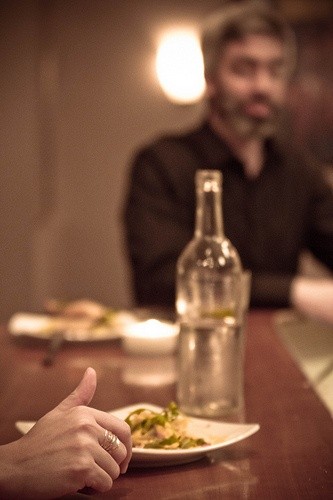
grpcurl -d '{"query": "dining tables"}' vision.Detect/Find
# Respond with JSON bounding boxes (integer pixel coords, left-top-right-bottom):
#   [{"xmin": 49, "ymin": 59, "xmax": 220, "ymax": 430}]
[{"xmin": 0, "ymin": 305, "xmax": 333, "ymax": 500}]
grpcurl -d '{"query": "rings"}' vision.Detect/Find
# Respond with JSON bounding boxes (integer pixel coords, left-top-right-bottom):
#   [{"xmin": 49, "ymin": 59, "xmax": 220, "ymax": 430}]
[{"xmin": 100, "ymin": 430, "xmax": 120, "ymax": 453}]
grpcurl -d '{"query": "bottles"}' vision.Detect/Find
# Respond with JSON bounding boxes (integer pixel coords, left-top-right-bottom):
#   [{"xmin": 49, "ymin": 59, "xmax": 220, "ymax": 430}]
[{"xmin": 175, "ymin": 169, "xmax": 244, "ymax": 427}]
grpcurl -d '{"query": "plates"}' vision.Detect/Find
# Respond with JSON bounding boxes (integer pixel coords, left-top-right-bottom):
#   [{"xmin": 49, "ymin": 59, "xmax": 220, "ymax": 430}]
[
  {"xmin": 8, "ymin": 313, "xmax": 120, "ymax": 343},
  {"xmin": 14, "ymin": 402, "xmax": 260, "ymax": 469}
]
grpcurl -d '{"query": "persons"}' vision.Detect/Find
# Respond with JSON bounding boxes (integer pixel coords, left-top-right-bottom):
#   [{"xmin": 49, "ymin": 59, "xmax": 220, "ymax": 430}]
[
  {"xmin": 0, "ymin": 366, "xmax": 132, "ymax": 500},
  {"xmin": 121, "ymin": 0, "xmax": 333, "ymax": 323}
]
